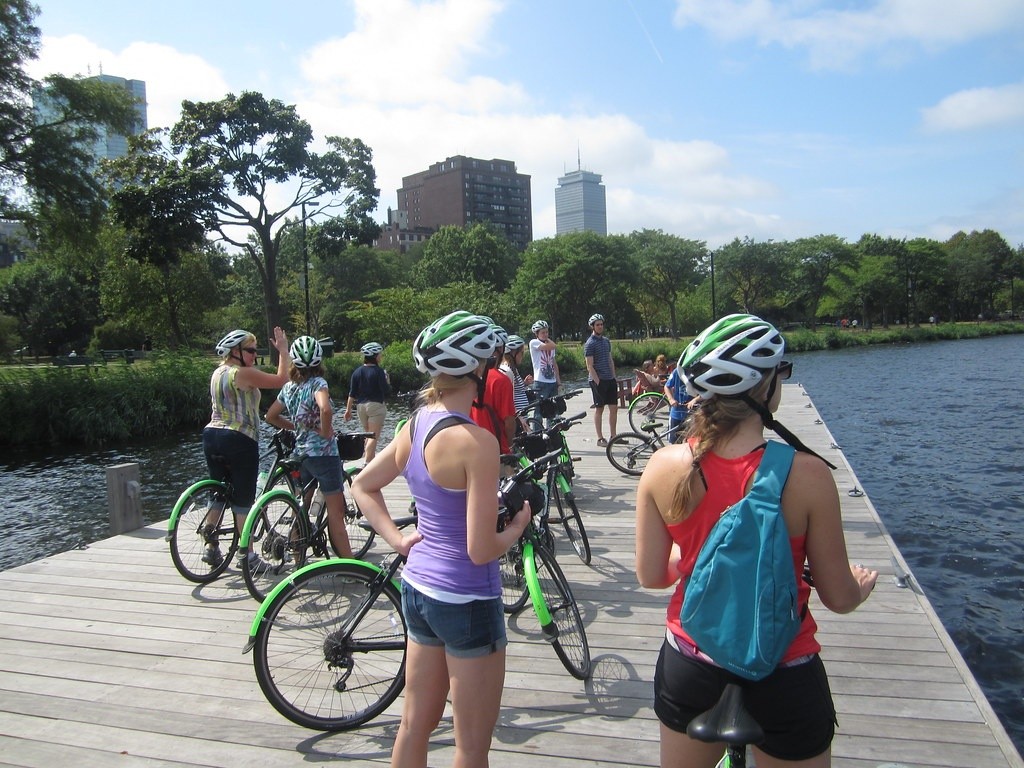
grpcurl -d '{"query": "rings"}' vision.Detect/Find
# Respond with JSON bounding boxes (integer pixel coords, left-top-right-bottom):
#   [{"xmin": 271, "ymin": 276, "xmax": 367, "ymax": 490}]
[{"xmin": 855, "ymin": 563, "xmax": 863, "ymax": 568}]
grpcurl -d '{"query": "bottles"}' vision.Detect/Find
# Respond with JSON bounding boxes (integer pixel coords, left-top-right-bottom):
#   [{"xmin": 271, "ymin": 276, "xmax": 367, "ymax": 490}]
[
  {"xmin": 537, "ymin": 482, "xmax": 548, "ymax": 517},
  {"xmin": 254, "ymin": 472, "xmax": 267, "ymax": 502},
  {"xmin": 310, "ymin": 485, "xmax": 325, "ymax": 516}
]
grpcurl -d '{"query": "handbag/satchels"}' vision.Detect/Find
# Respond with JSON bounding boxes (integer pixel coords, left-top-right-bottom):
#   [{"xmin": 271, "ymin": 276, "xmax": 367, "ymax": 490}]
[{"xmin": 679, "ymin": 439, "xmax": 807, "ymax": 681}]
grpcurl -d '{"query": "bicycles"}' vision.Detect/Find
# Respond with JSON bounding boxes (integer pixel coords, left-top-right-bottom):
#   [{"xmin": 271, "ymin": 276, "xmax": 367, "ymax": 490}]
[
  {"xmin": 686, "ymin": 561, "xmax": 876, "ymax": 768},
  {"xmin": 165, "ymin": 416, "xmax": 303, "ymax": 585},
  {"xmin": 627, "ymin": 373, "xmax": 673, "ymax": 439},
  {"xmin": 606, "ymin": 402, "xmax": 702, "ymax": 476},
  {"xmin": 236, "ymin": 430, "xmax": 377, "ymax": 605},
  {"xmin": 496, "ymin": 390, "xmax": 592, "ymax": 613},
  {"xmin": 241, "ymin": 446, "xmax": 592, "ymax": 733}
]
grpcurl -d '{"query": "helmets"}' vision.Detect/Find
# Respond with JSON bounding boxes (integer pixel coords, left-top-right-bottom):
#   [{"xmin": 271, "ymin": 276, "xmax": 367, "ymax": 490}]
[
  {"xmin": 532, "ymin": 320, "xmax": 548, "ymax": 333},
  {"xmin": 215, "ymin": 330, "xmax": 248, "ymax": 356},
  {"xmin": 505, "ymin": 334, "xmax": 525, "ymax": 349},
  {"xmin": 491, "ymin": 326, "xmax": 507, "ymax": 347},
  {"xmin": 588, "ymin": 314, "xmax": 604, "ymax": 327},
  {"xmin": 289, "ymin": 335, "xmax": 323, "ymax": 368},
  {"xmin": 412, "ymin": 310, "xmax": 495, "ymax": 376},
  {"xmin": 677, "ymin": 313, "xmax": 784, "ymax": 398},
  {"xmin": 360, "ymin": 342, "xmax": 383, "ymax": 356}
]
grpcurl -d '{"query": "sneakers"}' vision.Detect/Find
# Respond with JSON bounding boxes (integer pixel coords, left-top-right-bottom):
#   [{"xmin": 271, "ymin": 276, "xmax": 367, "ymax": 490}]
[
  {"xmin": 236, "ymin": 552, "xmax": 272, "ymax": 572},
  {"xmin": 597, "ymin": 439, "xmax": 609, "ymax": 447},
  {"xmin": 609, "ymin": 437, "xmax": 628, "ymax": 444},
  {"xmin": 201, "ymin": 548, "xmax": 223, "ymax": 565}
]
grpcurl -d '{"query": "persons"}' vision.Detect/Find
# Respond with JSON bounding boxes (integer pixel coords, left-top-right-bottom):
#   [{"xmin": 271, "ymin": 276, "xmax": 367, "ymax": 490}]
[
  {"xmin": 634, "ymin": 312, "xmax": 878, "ymax": 768},
  {"xmin": 200, "ymin": 327, "xmax": 291, "ymax": 573},
  {"xmin": 928, "ymin": 315, "xmax": 934, "ymax": 325},
  {"xmin": 350, "ymin": 309, "xmax": 531, "ymax": 768},
  {"xmin": 528, "ymin": 320, "xmax": 563, "ymax": 434},
  {"xmin": 852, "ymin": 318, "xmax": 858, "ymax": 328},
  {"xmin": 583, "ymin": 314, "xmax": 629, "ymax": 446},
  {"xmin": 836, "ymin": 318, "xmax": 850, "ymax": 329},
  {"xmin": 343, "ymin": 341, "xmax": 391, "ymax": 469},
  {"xmin": 263, "ymin": 336, "xmax": 372, "ymax": 584},
  {"xmin": 643, "ymin": 354, "xmax": 675, "ymax": 393},
  {"xmin": 663, "ymin": 368, "xmax": 704, "ymax": 446},
  {"xmin": 469, "ymin": 326, "xmax": 552, "ymax": 560}
]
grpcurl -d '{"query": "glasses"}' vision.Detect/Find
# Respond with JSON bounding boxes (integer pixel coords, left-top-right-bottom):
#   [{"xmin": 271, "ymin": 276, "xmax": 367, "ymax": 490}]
[
  {"xmin": 776, "ymin": 361, "xmax": 793, "ymax": 379},
  {"xmin": 238, "ymin": 348, "xmax": 256, "ymax": 354}
]
